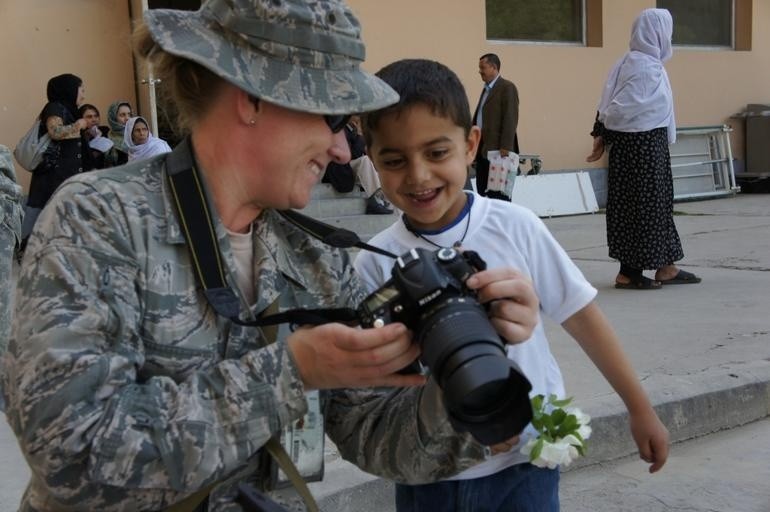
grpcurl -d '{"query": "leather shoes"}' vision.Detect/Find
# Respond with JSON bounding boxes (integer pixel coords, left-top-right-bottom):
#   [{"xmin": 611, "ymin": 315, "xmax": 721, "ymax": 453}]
[{"xmin": 366, "ymin": 204, "xmax": 393, "ymax": 214}]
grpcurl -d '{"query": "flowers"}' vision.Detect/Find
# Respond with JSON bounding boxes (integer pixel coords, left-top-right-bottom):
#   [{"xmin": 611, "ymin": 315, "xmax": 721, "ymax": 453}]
[{"xmin": 512, "ymin": 390, "xmax": 594, "ymax": 472}]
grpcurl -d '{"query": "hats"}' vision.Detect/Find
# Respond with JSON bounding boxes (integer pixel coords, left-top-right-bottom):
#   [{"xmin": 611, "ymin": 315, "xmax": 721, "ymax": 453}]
[{"xmin": 143, "ymin": 1, "xmax": 400, "ymax": 116}]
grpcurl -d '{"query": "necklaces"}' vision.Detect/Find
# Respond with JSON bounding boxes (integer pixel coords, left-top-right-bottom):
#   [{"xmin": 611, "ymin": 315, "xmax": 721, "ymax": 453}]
[{"xmin": 404, "ymin": 192, "xmax": 472, "ymax": 249}]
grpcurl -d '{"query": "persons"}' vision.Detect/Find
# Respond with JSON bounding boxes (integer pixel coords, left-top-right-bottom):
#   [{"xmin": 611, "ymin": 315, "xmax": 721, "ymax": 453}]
[
  {"xmin": 79, "ymin": 101, "xmax": 171, "ymax": 173},
  {"xmin": 28, "ymin": 73, "xmax": 91, "ymax": 217},
  {"xmin": 350, "ymin": 55, "xmax": 670, "ymax": 512},
  {"xmin": 322, "ymin": 115, "xmax": 394, "ymax": 216},
  {"xmin": 586, "ymin": 7, "xmax": 702, "ymax": 289},
  {"xmin": 3, "ymin": 1, "xmax": 540, "ymax": 512},
  {"xmin": 472, "ymin": 53, "xmax": 521, "ymax": 203}
]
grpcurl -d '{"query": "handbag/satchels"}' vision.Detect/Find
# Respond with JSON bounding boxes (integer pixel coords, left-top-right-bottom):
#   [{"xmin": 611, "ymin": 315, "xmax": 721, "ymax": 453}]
[
  {"xmin": 322, "ymin": 161, "xmax": 354, "ymax": 193},
  {"xmin": 13, "ymin": 117, "xmax": 51, "ymax": 171},
  {"xmin": 487, "ymin": 149, "xmax": 520, "ymax": 197}
]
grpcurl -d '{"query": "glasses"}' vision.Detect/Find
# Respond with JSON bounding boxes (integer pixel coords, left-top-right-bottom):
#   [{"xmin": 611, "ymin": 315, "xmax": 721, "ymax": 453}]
[{"xmin": 324, "ymin": 113, "xmax": 350, "ymax": 134}]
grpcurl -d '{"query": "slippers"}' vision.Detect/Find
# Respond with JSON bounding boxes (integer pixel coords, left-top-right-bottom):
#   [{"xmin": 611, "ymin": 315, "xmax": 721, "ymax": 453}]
[
  {"xmin": 614, "ymin": 277, "xmax": 662, "ymax": 289},
  {"xmin": 661, "ymin": 270, "xmax": 701, "ymax": 284}
]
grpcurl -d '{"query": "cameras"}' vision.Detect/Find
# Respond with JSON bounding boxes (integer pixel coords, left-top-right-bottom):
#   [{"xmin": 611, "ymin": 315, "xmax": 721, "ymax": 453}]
[{"xmin": 352, "ymin": 248, "xmax": 533, "ymax": 446}]
